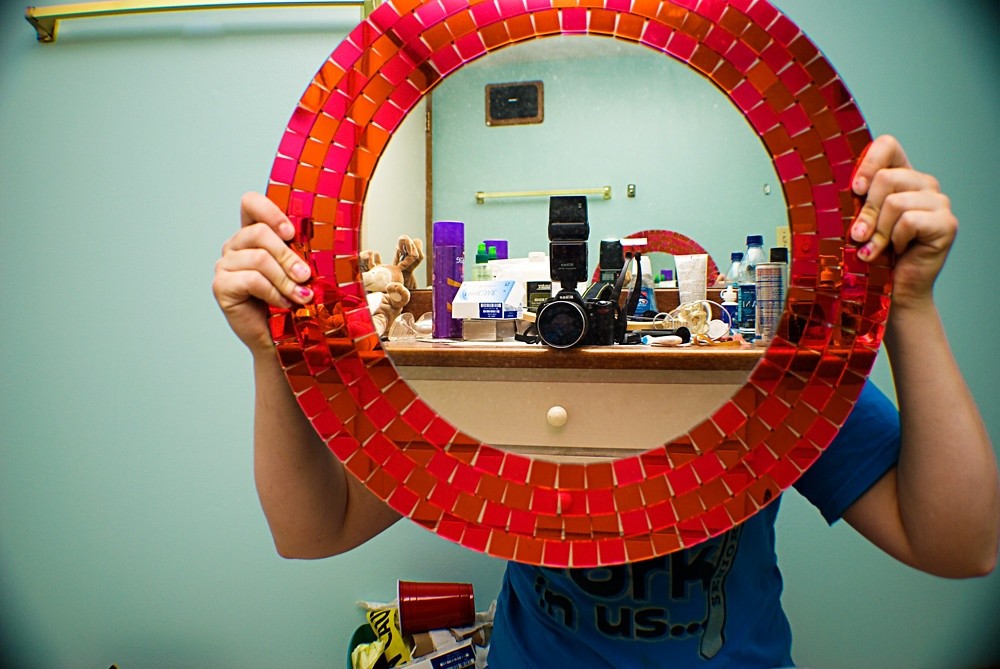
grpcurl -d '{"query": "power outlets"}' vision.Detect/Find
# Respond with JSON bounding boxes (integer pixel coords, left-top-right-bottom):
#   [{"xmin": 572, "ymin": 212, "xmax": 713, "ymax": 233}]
[{"xmin": 777, "ymin": 226, "xmax": 792, "ymax": 251}]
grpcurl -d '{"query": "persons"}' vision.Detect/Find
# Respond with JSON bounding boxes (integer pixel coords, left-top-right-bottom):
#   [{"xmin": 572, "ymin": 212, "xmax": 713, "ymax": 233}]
[{"xmin": 213, "ymin": 136, "xmax": 1000, "ymax": 669}]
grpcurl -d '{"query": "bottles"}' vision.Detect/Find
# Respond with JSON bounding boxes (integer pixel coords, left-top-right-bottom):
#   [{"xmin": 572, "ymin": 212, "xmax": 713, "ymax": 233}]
[
  {"xmin": 627, "ymin": 256, "xmax": 658, "ymax": 317},
  {"xmin": 654, "ymin": 269, "xmax": 673, "ymax": 288},
  {"xmin": 471, "ymin": 240, "xmax": 509, "ymax": 281},
  {"xmin": 432, "ymin": 222, "xmax": 465, "ymax": 339},
  {"xmin": 525, "ymin": 252, "xmax": 552, "ymax": 313},
  {"xmin": 721, "ymin": 251, "xmax": 744, "ymax": 336},
  {"xmin": 738, "ymin": 235, "xmax": 768, "ymax": 343}
]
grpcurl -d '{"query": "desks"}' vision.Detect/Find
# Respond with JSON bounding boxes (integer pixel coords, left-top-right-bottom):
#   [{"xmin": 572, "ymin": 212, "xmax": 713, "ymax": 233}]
[{"xmin": 380, "ymin": 291, "xmax": 769, "ymax": 462}]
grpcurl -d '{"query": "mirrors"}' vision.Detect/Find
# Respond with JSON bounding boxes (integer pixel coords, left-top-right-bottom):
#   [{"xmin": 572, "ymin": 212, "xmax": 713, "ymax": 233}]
[
  {"xmin": 355, "ymin": 32, "xmax": 789, "ymax": 289},
  {"xmin": 263, "ymin": 0, "xmax": 896, "ymax": 569},
  {"xmin": 592, "ymin": 230, "xmax": 720, "ymax": 288}
]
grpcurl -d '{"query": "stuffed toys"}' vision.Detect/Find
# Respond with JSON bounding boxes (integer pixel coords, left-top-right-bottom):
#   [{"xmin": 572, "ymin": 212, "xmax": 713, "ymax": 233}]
[{"xmin": 358, "ymin": 236, "xmax": 420, "ymax": 340}]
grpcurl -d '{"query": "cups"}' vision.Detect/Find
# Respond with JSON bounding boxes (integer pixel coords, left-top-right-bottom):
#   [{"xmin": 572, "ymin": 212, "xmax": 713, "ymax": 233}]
[{"xmin": 396, "ymin": 579, "xmax": 475, "ymax": 638}]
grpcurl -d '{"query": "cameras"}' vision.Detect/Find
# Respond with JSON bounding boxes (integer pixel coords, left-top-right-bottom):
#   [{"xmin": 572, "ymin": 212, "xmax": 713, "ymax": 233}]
[{"xmin": 535, "ymin": 196, "xmax": 623, "ymax": 349}]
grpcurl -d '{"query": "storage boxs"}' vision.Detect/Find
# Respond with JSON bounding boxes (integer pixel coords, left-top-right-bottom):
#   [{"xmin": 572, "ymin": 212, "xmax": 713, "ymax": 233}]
[
  {"xmin": 452, "ymin": 280, "xmax": 519, "ymax": 320},
  {"xmin": 389, "ymin": 637, "xmax": 477, "ymax": 669}
]
